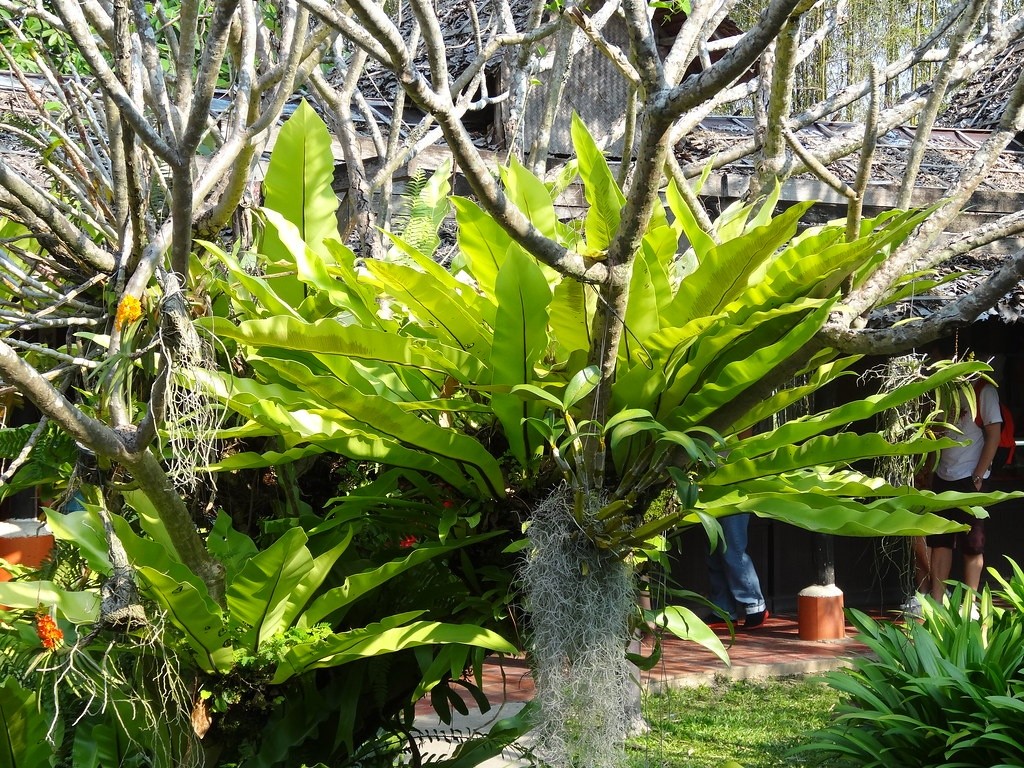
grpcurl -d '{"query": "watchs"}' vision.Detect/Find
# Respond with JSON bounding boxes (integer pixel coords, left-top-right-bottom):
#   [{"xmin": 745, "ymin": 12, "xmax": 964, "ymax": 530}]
[{"xmin": 972, "ymin": 474, "xmax": 982, "ymax": 483}]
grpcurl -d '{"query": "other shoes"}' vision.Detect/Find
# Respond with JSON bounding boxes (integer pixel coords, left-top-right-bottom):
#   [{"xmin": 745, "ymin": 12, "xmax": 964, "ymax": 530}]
[
  {"xmin": 899, "ymin": 594, "xmax": 926, "ymax": 609},
  {"xmin": 702, "ymin": 613, "xmax": 738, "ymax": 628},
  {"xmin": 743, "ymin": 609, "xmax": 769, "ymax": 630},
  {"xmin": 909, "ymin": 589, "xmax": 952, "ymax": 615}
]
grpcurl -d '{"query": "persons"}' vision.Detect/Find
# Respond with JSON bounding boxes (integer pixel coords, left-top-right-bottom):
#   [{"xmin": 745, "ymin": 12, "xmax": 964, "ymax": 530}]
[
  {"xmin": 930, "ymin": 337, "xmax": 1003, "ymax": 622},
  {"xmin": 701, "ymin": 425, "xmax": 769, "ymax": 630},
  {"xmin": 900, "ymin": 536, "xmax": 931, "ymax": 617}
]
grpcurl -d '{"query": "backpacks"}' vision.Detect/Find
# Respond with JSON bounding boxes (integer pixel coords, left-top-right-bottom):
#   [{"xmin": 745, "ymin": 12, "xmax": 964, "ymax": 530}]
[{"xmin": 974, "ymin": 379, "xmax": 1015, "ymax": 472}]
